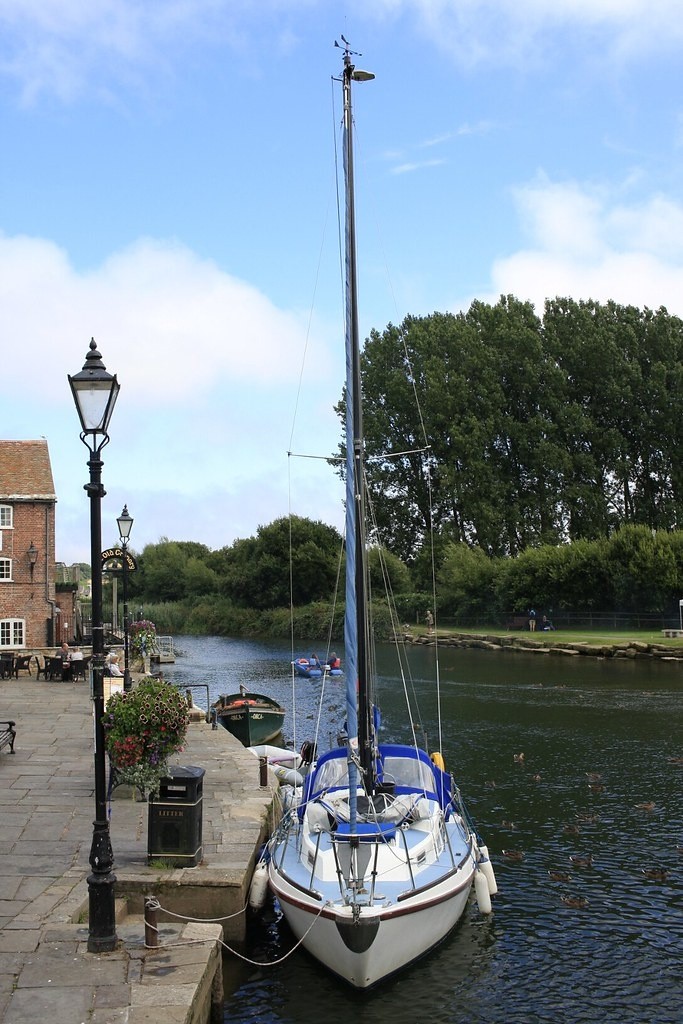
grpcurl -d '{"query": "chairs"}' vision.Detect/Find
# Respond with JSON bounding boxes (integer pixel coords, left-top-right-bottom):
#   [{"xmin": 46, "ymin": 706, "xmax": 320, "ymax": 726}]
[
  {"xmin": 35, "ymin": 655, "xmax": 93, "ymax": 683},
  {"xmin": 0, "ymin": 653, "xmax": 33, "ymax": 679}
]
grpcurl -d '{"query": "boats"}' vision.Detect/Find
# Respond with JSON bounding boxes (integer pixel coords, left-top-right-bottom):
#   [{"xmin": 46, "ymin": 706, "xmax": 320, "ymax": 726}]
[
  {"xmin": 291, "ymin": 657, "xmax": 323, "ymax": 678},
  {"xmin": 211, "ymin": 685, "xmax": 287, "ymax": 746},
  {"xmin": 319, "ymin": 660, "xmax": 343, "ymax": 675}
]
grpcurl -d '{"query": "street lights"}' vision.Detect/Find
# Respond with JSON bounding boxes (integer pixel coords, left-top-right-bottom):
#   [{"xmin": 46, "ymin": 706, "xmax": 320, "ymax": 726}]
[
  {"xmin": 114, "ymin": 504, "xmax": 133, "ymax": 692},
  {"xmin": 66, "ymin": 335, "xmax": 122, "ymax": 953}
]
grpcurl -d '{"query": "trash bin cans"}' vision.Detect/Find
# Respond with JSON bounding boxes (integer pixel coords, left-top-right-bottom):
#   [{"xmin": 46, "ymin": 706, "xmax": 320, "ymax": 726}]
[{"xmin": 146, "ymin": 765, "xmax": 206, "ymax": 868}]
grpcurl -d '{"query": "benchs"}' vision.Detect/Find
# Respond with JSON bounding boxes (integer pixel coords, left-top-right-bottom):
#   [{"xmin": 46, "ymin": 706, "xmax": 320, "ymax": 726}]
[{"xmin": 0, "ymin": 721, "xmax": 16, "ymax": 754}]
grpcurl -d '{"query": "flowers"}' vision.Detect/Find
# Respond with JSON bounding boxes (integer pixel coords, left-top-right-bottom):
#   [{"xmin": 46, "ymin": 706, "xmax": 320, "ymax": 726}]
[
  {"xmin": 99, "ymin": 676, "xmax": 191, "ymax": 802},
  {"xmin": 129, "ymin": 619, "xmax": 156, "ymax": 647}
]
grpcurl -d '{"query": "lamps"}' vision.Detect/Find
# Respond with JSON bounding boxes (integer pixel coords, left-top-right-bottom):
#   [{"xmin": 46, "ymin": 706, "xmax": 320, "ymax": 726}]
[{"xmin": 27, "ymin": 541, "xmax": 39, "ymax": 568}]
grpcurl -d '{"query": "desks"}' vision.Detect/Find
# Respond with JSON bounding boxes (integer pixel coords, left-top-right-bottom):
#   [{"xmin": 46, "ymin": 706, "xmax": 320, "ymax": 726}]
[
  {"xmin": 0, "ymin": 658, "xmax": 12, "ymax": 678},
  {"xmin": 61, "ymin": 660, "xmax": 74, "ymax": 683}
]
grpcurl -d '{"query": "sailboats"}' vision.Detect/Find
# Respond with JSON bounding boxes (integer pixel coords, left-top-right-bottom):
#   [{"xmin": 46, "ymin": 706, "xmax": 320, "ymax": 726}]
[{"xmin": 261, "ymin": 33, "xmax": 497, "ymax": 989}]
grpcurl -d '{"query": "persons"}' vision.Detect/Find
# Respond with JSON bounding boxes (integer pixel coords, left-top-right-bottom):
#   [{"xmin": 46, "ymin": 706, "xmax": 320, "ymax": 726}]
[
  {"xmin": 425, "ymin": 611, "xmax": 434, "ymax": 635},
  {"xmin": 324, "ymin": 652, "xmax": 340, "ymax": 670},
  {"xmin": 527, "ymin": 607, "xmax": 536, "ymax": 631},
  {"xmin": 56, "ymin": 643, "xmax": 83, "ymax": 661},
  {"xmin": 105, "ymin": 653, "xmax": 135, "ymax": 682},
  {"xmin": 309, "ymin": 654, "xmax": 322, "ymax": 670}
]
